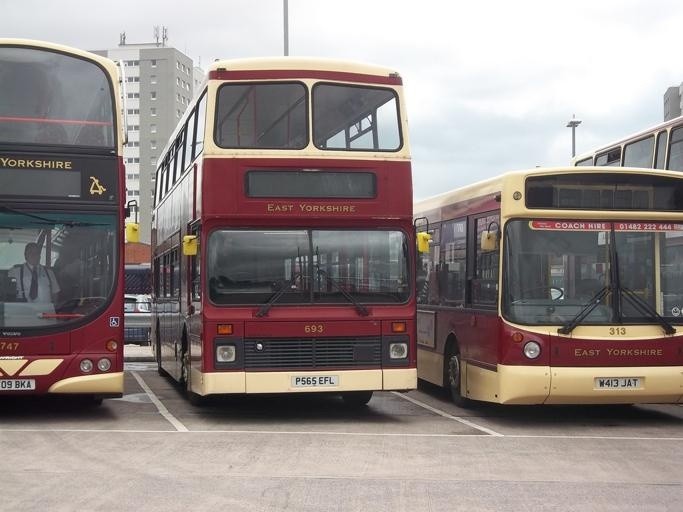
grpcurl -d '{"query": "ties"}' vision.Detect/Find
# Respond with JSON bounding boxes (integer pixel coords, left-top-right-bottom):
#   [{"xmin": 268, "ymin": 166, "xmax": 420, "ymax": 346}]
[{"xmin": 29, "ymin": 266, "xmax": 38, "ymax": 299}]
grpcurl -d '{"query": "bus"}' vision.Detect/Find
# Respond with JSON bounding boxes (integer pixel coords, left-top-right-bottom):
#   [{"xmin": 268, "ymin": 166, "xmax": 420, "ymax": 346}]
[
  {"xmin": 1, "ymin": 37, "xmax": 140, "ymax": 406},
  {"xmin": 563, "ymin": 116, "xmax": 683, "ymax": 310},
  {"xmin": 149, "ymin": 56, "xmax": 432, "ymax": 406},
  {"xmin": 392, "ymin": 165, "xmax": 682, "ymax": 409}
]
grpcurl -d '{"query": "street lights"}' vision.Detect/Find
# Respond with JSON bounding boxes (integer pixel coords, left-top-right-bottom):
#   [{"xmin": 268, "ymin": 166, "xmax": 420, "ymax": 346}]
[{"xmin": 565, "ymin": 114, "xmax": 582, "ymax": 158}]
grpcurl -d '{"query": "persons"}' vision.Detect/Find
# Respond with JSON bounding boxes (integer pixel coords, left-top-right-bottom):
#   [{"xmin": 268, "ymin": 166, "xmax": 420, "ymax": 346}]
[{"xmin": 4, "ymin": 242, "xmax": 64, "ymax": 310}]
